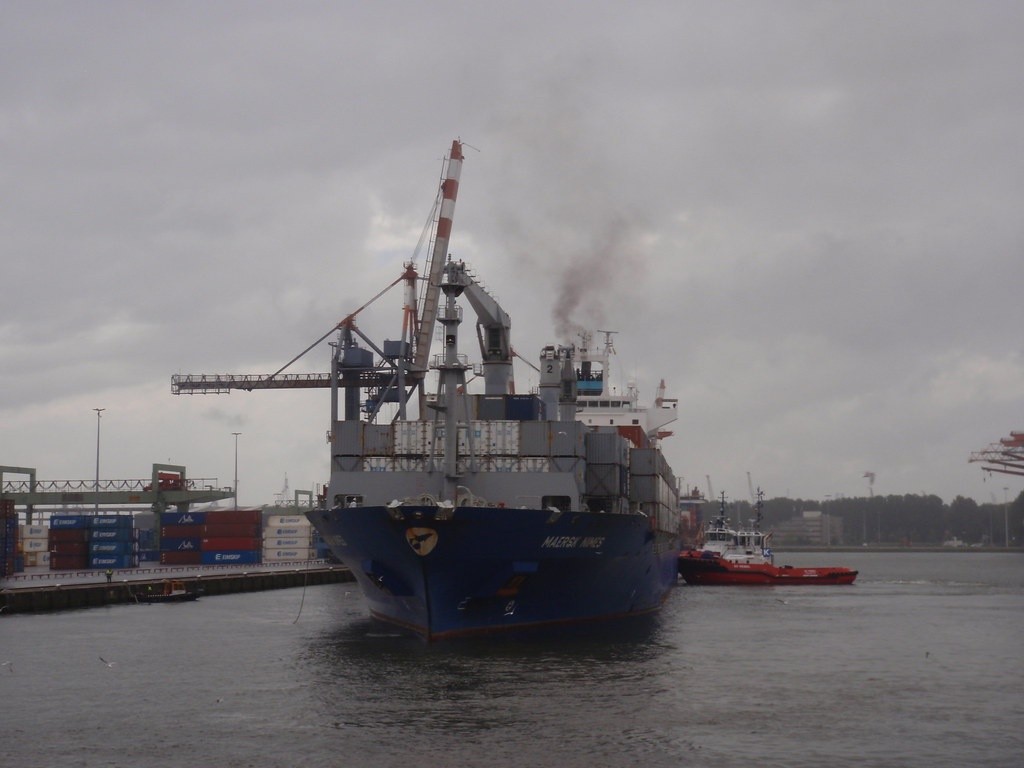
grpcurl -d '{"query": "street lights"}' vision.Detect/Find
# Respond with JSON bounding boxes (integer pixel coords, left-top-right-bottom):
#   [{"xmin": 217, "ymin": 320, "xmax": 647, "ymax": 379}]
[
  {"xmin": 232, "ymin": 431, "xmax": 242, "ymax": 511},
  {"xmin": 824, "ymin": 494, "xmax": 832, "ymax": 546},
  {"xmin": 93, "ymin": 407, "xmax": 107, "ymax": 516},
  {"xmin": 1003, "ymin": 487, "xmax": 1010, "ymax": 551}
]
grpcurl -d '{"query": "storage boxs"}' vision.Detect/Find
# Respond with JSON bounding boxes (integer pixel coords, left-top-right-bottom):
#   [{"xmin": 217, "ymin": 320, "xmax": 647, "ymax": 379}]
[
  {"xmin": 0, "ymin": 498, "xmax": 329, "ymax": 575},
  {"xmin": 331, "ymin": 395, "xmax": 680, "ymax": 533}
]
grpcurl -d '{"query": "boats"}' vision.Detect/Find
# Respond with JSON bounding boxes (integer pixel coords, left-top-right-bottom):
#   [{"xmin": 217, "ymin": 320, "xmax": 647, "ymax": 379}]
[
  {"xmin": 699, "ymin": 489, "xmax": 738, "ymax": 559},
  {"xmin": 144, "ymin": 582, "xmax": 200, "ymax": 604},
  {"xmin": 301, "ymin": 260, "xmax": 690, "ymax": 644},
  {"xmin": 721, "ymin": 485, "xmax": 775, "ymax": 567},
  {"xmin": 677, "ymin": 548, "xmax": 860, "ymax": 586}
]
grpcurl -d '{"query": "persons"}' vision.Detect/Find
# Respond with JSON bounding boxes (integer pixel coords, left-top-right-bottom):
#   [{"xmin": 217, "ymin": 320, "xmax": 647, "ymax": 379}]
[
  {"xmin": 146, "ymin": 585, "xmax": 152, "ymax": 594},
  {"xmin": 105, "ymin": 568, "xmax": 113, "ymax": 583},
  {"xmin": 349, "ymin": 498, "xmax": 356, "ymax": 507}
]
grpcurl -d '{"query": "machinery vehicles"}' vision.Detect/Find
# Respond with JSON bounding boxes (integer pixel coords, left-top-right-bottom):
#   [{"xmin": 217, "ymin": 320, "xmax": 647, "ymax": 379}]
[{"xmin": 171, "ymin": 139, "xmax": 548, "ymax": 421}]
